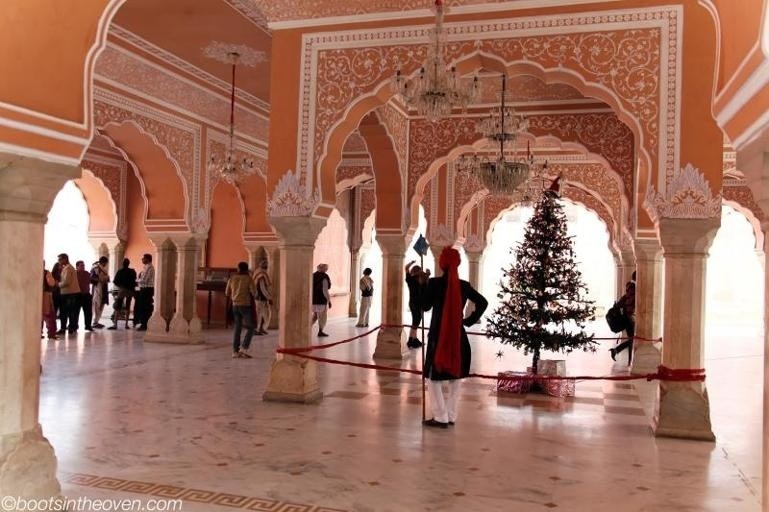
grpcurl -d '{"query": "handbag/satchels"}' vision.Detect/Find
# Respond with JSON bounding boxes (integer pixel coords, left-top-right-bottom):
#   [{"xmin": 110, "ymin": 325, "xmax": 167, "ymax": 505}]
[
  {"xmin": 113, "ymin": 298, "xmax": 122, "ymax": 310},
  {"xmin": 90, "ymin": 272, "xmax": 99, "ymax": 284}
]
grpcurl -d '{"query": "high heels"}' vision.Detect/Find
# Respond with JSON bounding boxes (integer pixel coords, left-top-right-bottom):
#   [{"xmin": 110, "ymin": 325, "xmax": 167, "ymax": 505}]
[{"xmin": 608, "ymin": 347, "xmax": 616, "ymax": 362}]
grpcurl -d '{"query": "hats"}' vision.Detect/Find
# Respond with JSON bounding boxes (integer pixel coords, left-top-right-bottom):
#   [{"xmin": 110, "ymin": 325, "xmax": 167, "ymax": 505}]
[
  {"xmin": 259, "ymin": 260, "xmax": 268, "ymax": 267},
  {"xmin": 317, "ymin": 264, "xmax": 328, "ymax": 272}
]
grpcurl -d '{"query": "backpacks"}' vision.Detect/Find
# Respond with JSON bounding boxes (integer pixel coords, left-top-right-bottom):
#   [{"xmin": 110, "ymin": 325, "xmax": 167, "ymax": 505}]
[{"xmin": 606, "ymin": 295, "xmax": 628, "ymax": 333}]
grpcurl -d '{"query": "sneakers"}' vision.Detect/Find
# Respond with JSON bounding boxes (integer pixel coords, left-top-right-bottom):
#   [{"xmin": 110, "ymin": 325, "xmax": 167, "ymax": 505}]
[
  {"xmin": 41, "ymin": 322, "xmax": 149, "ymax": 339},
  {"xmin": 254, "ymin": 329, "xmax": 268, "ymax": 335},
  {"xmin": 239, "ymin": 347, "xmax": 253, "ymax": 358},
  {"xmin": 231, "ymin": 351, "xmax": 241, "ymax": 358}
]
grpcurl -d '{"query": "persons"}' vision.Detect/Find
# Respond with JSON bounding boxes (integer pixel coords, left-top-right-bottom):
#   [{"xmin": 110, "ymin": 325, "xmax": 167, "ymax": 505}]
[
  {"xmin": 416, "ymin": 245, "xmax": 488, "ymax": 431},
  {"xmin": 606, "ymin": 270, "xmax": 636, "ymax": 367},
  {"xmin": 57, "ymin": 253, "xmax": 82, "ymax": 334},
  {"xmin": 224, "ymin": 260, "xmax": 258, "ymax": 360},
  {"xmin": 133, "ymin": 252, "xmax": 156, "ymax": 328},
  {"xmin": 41, "ymin": 259, "xmax": 60, "ymax": 339},
  {"xmin": 311, "ymin": 262, "xmax": 333, "ymax": 338},
  {"xmin": 107, "ymin": 257, "xmax": 137, "ymax": 331},
  {"xmin": 252, "ymin": 258, "xmax": 275, "ymax": 336},
  {"xmin": 404, "ymin": 258, "xmax": 431, "ymax": 349},
  {"xmin": 74, "ymin": 260, "xmax": 96, "ymax": 331},
  {"xmin": 51, "ymin": 263, "xmax": 62, "ymax": 315},
  {"xmin": 88, "ymin": 256, "xmax": 112, "ymax": 329},
  {"xmin": 355, "ymin": 267, "xmax": 374, "ymax": 329}
]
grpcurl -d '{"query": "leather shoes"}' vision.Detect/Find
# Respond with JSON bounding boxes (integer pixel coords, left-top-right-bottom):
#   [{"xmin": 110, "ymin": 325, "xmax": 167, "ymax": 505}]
[
  {"xmin": 424, "ymin": 418, "xmax": 448, "ymax": 428},
  {"xmin": 318, "ymin": 332, "xmax": 328, "ymax": 336},
  {"xmin": 407, "ymin": 336, "xmax": 425, "ymax": 348}
]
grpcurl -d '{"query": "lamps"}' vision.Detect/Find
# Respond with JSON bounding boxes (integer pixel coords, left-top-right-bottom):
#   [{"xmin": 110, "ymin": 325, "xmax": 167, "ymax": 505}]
[
  {"xmin": 208, "ymin": 50, "xmax": 257, "ymax": 184},
  {"xmin": 391, "ymin": 0, "xmax": 483, "ymax": 127},
  {"xmin": 456, "ymin": 65, "xmax": 548, "ymax": 197}
]
